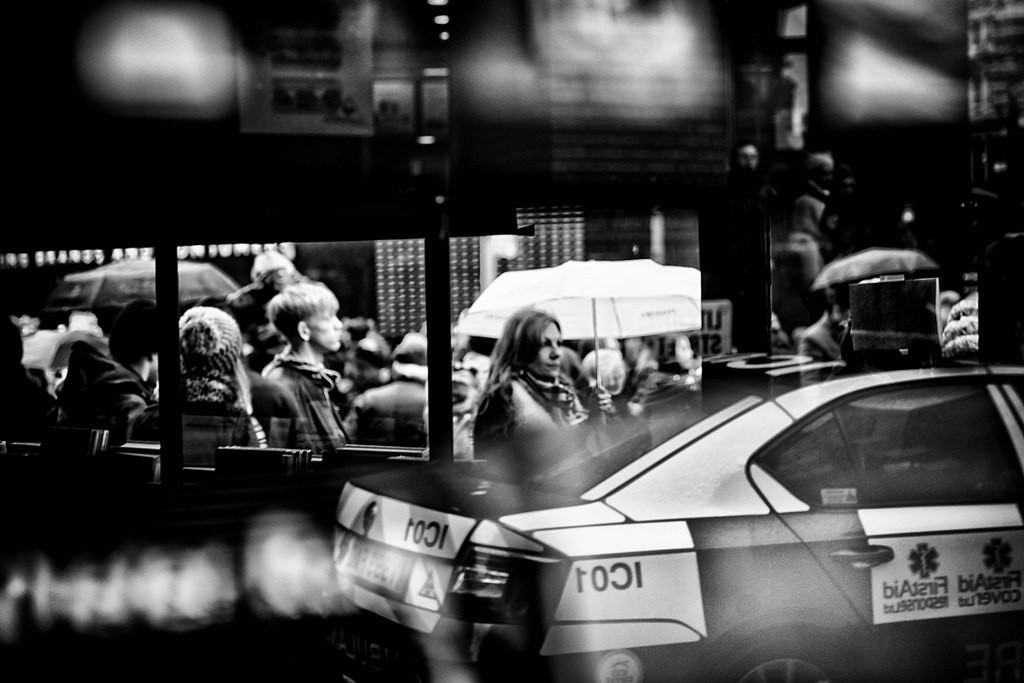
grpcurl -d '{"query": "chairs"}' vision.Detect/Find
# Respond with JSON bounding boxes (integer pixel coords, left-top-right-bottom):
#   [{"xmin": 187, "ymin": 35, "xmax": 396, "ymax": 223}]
[{"xmin": 886, "ymin": 407, "xmax": 979, "ymax": 502}]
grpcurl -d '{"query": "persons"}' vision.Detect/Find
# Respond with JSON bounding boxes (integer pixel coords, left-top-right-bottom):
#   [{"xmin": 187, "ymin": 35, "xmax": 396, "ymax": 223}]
[
  {"xmin": 1, "ymin": 250, "xmax": 705, "ymax": 470},
  {"xmin": 743, "ymin": 147, "xmax": 979, "ymax": 391}
]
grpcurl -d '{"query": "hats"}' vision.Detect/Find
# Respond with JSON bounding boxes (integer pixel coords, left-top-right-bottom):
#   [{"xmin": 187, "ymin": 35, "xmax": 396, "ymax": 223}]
[
  {"xmin": 179, "ymin": 305, "xmax": 241, "ymax": 372},
  {"xmin": 252, "ymin": 251, "xmax": 293, "ymax": 281}
]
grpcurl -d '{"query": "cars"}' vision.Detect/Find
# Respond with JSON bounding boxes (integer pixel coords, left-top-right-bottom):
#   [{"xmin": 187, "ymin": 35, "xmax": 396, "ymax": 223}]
[{"xmin": 330, "ymin": 361, "xmax": 1023, "ymax": 683}]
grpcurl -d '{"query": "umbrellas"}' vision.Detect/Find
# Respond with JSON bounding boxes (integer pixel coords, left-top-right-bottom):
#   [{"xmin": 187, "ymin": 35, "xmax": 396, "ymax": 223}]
[
  {"xmin": 45, "ymin": 261, "xmax": 241, "ymax": 312},
  {"xmin": 459, "ymin": 258, "xmax": 700, "ymax": 389},
  {"xmin": 810, "ymin": 247, "xmax": 937, "ymax": 291}
]
